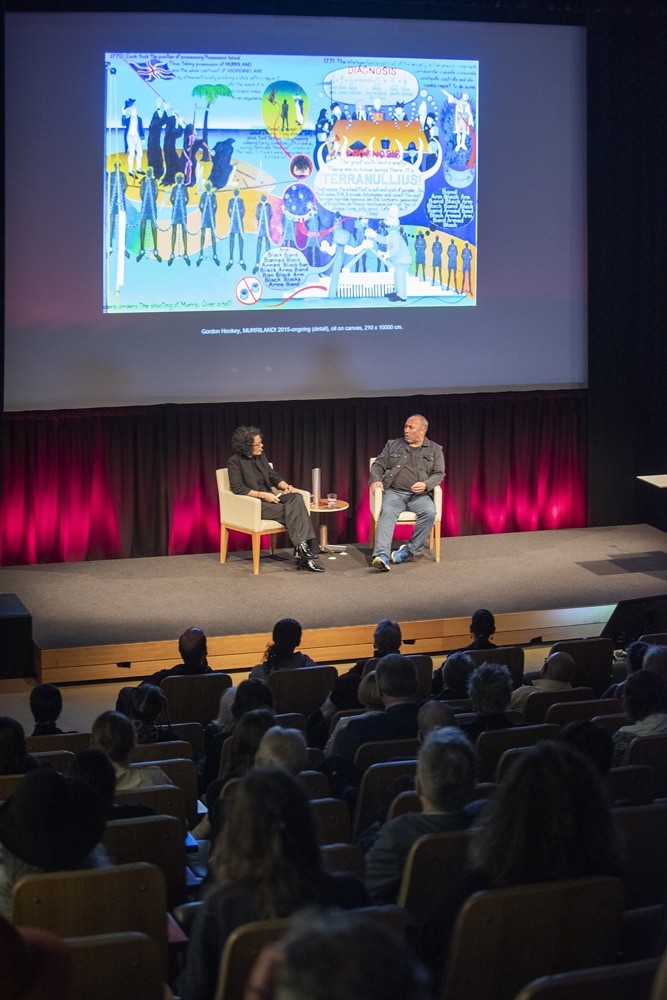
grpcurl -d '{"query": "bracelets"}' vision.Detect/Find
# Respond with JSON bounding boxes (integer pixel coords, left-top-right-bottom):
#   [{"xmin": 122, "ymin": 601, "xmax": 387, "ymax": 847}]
[{"xmin": 257, "ymin": 491, "xmax": 259, "ymax": 498}]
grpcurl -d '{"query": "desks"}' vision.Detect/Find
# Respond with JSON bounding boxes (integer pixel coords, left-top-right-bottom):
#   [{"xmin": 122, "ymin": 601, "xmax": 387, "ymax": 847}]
[
  {"xmin": 637, "ymin": 474, "xmax": 667, "ymax": 489},
  {"xmin": 310, "ymin": 500, "xmax": 350, "ymax": 557}
]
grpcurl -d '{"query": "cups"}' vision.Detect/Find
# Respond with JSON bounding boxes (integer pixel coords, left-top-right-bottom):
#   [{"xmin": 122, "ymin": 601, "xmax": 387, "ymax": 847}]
[{"xmin": 327, "ymin": 494, "xmax": 337, "ymax": 508}]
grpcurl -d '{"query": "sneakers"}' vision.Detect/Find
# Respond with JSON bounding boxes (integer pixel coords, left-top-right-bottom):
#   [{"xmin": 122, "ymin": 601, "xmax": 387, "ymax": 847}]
[
  {"xmin": 390, "ymin": 544, "xmax": 410, "ymax": 563},
  {"xmin": 372, "ymin": 554, "xmax": 391, "ymax": 572}
]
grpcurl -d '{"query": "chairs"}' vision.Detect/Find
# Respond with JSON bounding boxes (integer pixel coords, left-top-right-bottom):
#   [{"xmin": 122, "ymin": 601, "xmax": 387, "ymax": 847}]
[
  {"xmin": 215, "ymin": 463, "xmax": 311, "ymax": 575},
  {"xmin": 369, "ymin": 458, "xmax": 442, "ymax": 563},
  {"xmin": 0, "ymin": 631, "xmax": 667, "ymax": 1000}
]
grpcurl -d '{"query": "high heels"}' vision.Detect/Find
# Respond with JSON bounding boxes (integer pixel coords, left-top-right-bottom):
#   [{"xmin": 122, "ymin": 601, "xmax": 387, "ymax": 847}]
[
  {"xmin": 297, "ymin": 560, "xmax": 325, "ymax": 572},
  {"xmin": 293, "ymin": 543, "xmax": 319, "ymax": 559}
]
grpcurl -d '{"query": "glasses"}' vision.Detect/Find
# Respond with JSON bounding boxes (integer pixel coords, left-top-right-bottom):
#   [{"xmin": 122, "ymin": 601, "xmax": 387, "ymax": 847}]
[{"xmin": 252, "ymin": 442, "xmax": 264, "ymax": 446}]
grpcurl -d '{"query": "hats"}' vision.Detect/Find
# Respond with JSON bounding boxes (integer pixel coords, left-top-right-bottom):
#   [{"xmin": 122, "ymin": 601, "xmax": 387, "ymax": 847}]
[
  {"xmin": 1, "ymin": 769, "xmax": 108, "ymax": 870},
  {"xmin": 473, "ymin": 609, "xmax": 495, "ymax": 635}
]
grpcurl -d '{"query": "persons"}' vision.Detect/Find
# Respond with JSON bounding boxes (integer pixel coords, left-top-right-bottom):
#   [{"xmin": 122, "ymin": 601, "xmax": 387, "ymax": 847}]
[
  {"xmin": 227, "ymin": 427, "xmax": 326, "ymax": 573},
  {"xmin": 367, "ymin": 415, "xmax": 446, "ymax": 572},
  {"xmin": 0, "ymin": 608, "xmax": 666, "ymax": 1000}
]
